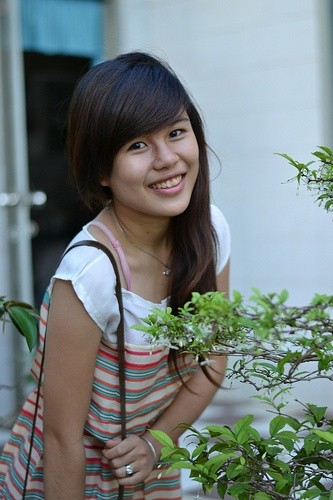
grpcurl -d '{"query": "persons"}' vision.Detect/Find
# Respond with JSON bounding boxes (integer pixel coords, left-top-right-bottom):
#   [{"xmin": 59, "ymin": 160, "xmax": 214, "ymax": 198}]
[{"xmin": 0, "ymin": 53, "xmax": 232, "ymax": 500}]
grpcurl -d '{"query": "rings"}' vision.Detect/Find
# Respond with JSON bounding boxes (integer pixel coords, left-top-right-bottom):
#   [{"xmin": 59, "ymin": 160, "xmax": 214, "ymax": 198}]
[{"xmin": 126, "ymin": 464, "xmax": 134, "ymax": 477}]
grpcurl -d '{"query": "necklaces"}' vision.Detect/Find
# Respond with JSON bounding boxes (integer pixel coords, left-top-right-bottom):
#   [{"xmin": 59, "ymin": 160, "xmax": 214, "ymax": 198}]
[{"xmin": 115, "ymin": 215, "xmax": 171, "ymax": 276}]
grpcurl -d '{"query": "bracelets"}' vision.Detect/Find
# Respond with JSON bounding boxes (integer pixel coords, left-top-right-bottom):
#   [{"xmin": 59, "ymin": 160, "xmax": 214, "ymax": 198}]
[{"xmin": 141, "ymin": 436, "xmax": 159, "ymax": 465}]
